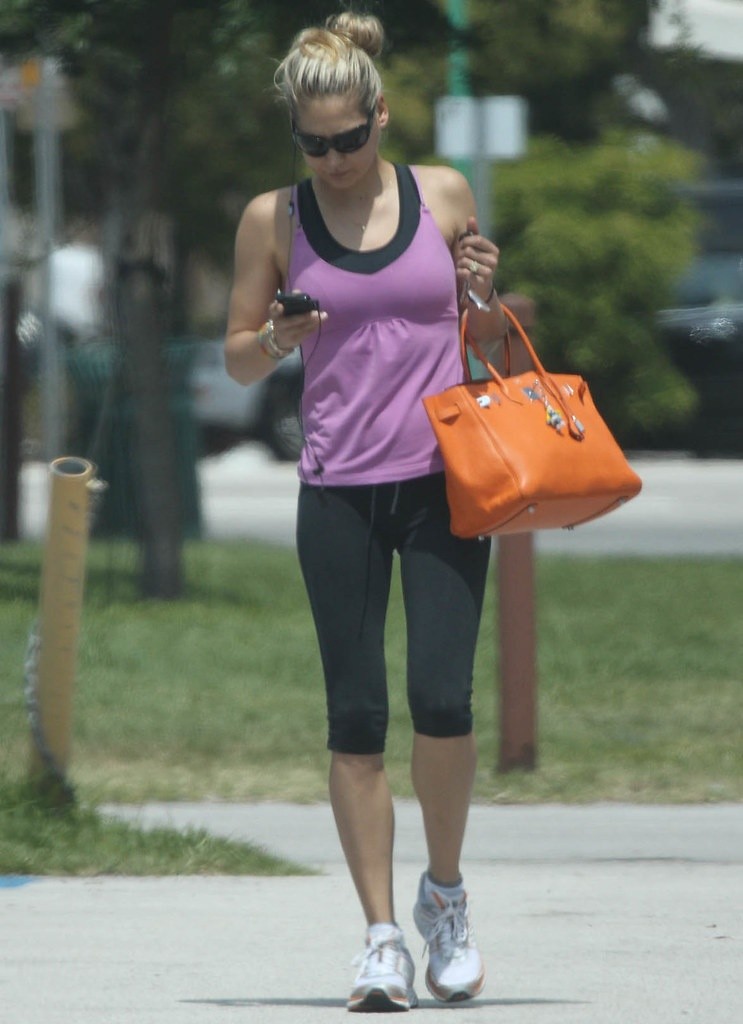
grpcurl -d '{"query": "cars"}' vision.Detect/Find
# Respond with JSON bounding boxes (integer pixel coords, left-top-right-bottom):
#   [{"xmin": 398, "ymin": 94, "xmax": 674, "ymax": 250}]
[{"xmin": 190, "ymin": 185, "xmax": 743, "ymax": 460}]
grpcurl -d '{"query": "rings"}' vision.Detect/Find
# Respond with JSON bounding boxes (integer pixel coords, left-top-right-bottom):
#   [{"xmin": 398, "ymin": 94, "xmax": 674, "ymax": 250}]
[{"xmin": 471, "ymin": 260, "xmax": 479, "ymax": 273}]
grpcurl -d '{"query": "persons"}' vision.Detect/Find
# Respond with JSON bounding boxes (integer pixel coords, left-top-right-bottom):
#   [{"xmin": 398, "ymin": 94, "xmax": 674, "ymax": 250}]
[{"xmin": 223, "ymin": 10, "xmax": 508, "ymax": 1012}]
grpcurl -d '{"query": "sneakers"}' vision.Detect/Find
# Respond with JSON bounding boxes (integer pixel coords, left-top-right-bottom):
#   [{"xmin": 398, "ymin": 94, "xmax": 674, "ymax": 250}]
[
  {"xmin": 346, "ymin": 929, "xmax": 419, "ymax": 1011},
  {"xmin": 411, "ymin": 870, "xmax": 485, "ymax": 1003}
]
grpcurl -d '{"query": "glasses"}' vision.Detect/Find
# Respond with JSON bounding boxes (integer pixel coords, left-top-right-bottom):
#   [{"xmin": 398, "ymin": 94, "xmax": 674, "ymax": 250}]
[{"xmin": 290, "ymin": 113, "xmax": 373, "ymax": 156}]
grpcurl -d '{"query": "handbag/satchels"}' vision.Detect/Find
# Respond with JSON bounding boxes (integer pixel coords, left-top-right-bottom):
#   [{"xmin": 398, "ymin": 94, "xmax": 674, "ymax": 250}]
[{"xmin": 422, "ymin": 299, "xmax": 641, "ymax": 536}]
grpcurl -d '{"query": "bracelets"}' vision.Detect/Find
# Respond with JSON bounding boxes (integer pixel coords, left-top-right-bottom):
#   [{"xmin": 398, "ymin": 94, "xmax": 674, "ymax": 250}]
[
  {"xmin": 258, "ymin": 318, "xmax": 294, "ymax": 360},
  {"xmin": 468, "ymin": 280, "xmax": 495, "ymax": 305}
]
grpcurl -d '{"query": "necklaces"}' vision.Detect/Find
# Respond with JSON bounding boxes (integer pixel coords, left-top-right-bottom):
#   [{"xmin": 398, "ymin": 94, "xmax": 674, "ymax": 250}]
[{"xmin": 362, "ymin": 225, "xmax": 366, "ymax": 231}]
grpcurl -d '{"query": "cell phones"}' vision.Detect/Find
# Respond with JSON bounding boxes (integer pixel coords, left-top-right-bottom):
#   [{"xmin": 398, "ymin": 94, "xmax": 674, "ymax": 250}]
[{"xmin": 276, "ymin": 294, "xmax": 316, "ymax": 315}]
